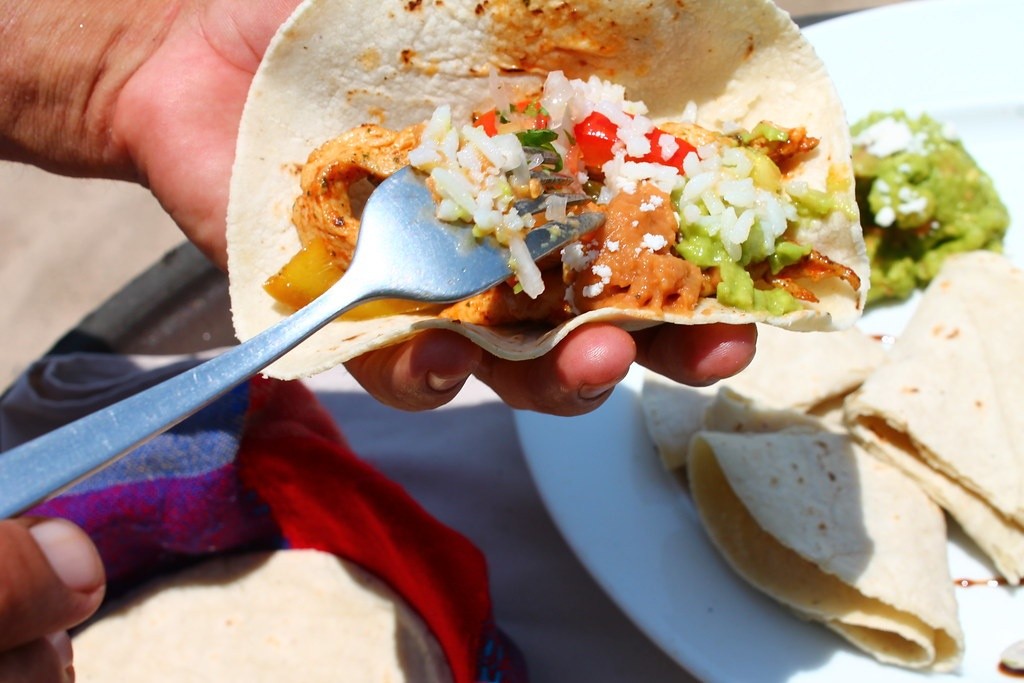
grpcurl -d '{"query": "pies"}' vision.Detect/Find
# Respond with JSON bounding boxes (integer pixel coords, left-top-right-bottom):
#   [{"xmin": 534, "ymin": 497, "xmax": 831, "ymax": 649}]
[
  {"xmin": 641, "ymin": 251, "xmax": 1024, "ymax": 673},
  {"xmin": 225, "ymin": 0, "xmax": 871, "ymax": 380}
]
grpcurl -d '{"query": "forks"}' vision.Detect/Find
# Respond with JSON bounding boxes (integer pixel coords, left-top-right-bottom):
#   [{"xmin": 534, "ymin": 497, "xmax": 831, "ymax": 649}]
[{"xmin": 0, "ymin": 146, "xmax": 606, "ymax": 523}]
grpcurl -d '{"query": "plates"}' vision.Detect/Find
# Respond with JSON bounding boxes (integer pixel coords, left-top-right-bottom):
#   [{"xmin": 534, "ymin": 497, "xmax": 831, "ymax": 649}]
[{"xmin": 513, "ymin": 0, "xmax": 1024, "ymax": 683}]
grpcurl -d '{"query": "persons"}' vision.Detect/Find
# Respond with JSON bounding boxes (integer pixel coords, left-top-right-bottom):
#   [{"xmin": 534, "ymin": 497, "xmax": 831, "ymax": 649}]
[{"xmin": 1, "ymin": 0, "xmax": 763, "ymax": 683}]
